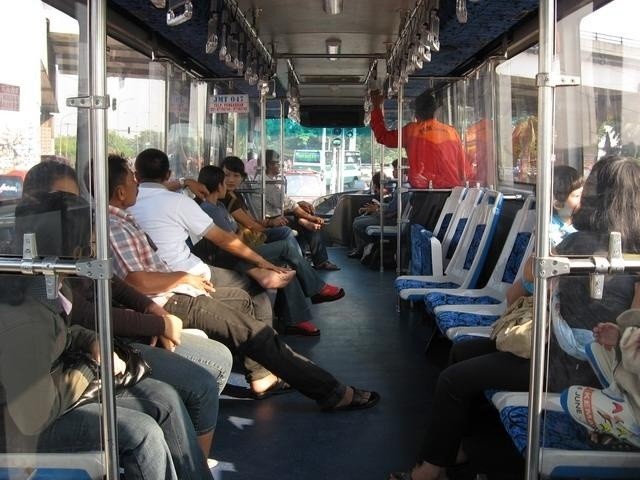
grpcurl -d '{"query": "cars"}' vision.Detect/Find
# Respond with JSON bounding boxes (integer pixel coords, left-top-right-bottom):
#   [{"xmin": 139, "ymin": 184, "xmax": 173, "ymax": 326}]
[{"xmin": 285, "ymin": 168, "xmax": 328, "ymax": 207}]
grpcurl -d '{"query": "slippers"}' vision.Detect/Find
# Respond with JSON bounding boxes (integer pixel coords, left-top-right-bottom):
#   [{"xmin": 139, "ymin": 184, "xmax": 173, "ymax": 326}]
[
  {"xmin": 311, "ymin": 284, "xmax": 344, "ymax": 304},
  {"xmin": 316, "ymin": 261, "xmax": 340, "ymax": 271},
  {"xmin": 321, "ymin": 386, "xmax": 379, "ymax": 410},
  {"xmin": 285, "ymin": 321, "xmax": 320, "ymax": 337},
  {"xmin": 254, "ymin": 377, "xmax": 295, "ymax": 399}
]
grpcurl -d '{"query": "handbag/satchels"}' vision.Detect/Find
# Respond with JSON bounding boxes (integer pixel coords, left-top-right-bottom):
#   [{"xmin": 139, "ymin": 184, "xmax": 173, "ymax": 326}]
[
  {"xmin": 60, "ymin": 335, "xmax": 151, "ymax": 416},
  {"xmin": 490, "ymin": 297, "xmax": 548, "ymax": 359},
  {"xmin": 361, "ymin": 239, "xmax": 397, "ymax": 270}
]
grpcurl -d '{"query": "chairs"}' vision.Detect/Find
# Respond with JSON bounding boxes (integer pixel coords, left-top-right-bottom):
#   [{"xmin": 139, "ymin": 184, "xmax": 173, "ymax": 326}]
[
  {"xmin": 0, "ymin": 448, "xmax": 107, "ymax": 480},
  {"xmin": 365, "ymin": 187, "xmax": 640, "ymax": 480},
  {"xmin": 182, "ymin": 324, "xmax": 210, "ymax": 341}
]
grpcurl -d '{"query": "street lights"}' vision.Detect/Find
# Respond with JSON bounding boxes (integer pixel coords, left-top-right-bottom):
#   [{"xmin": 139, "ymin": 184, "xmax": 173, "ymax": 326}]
[
  {"xmin": 64, "ymin": 121, "xmax": 76, "ymax": 158},
  {"xmin": 117, "ymin": 110, "xmax": 128, "ymax": 135},
  {"xmin": 58, "ymin": 108, "xmax": 77, "ymax": 155},
  {"xmin": 116, "ymin": 96, "xmax": 136, "ymax": 150}
]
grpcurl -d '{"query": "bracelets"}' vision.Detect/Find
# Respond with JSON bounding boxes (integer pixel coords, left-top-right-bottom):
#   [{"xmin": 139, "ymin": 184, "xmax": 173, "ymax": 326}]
[{"xmin": 179, "ymin": 178, "xmax": 185, "ymax": 188}]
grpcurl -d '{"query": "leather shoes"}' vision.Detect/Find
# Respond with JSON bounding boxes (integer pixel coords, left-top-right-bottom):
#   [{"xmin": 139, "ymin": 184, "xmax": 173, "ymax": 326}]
[{"xmin": 348, "ymin": 249, "xmax": 361, "ymax": 258}]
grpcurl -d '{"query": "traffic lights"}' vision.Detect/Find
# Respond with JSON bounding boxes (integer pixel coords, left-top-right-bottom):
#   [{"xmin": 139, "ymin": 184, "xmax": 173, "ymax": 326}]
[
  {"xmin": 127, "ymin": 126, "xmax": 131, "ymax": 135},
  {"xmin": 331, "ymin": 128, "xmax": 341, "ymax": 148},
  {"xmin": 345, "ymin": 128, "xmax": 353, "ymax": 138}
]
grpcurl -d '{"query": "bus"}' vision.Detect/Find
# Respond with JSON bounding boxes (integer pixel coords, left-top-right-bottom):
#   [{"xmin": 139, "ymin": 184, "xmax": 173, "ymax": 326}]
[{"xmin": 291, "ymin": 148, "xmax": 363, "ymax": 188}]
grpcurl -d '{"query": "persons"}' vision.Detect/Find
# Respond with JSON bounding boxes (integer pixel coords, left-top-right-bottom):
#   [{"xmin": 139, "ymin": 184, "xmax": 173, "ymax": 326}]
[
  {"xmin": 83, "ymin": 154, "xmax": 382, "ymax": 409},
  {"xmin": 128, "ymin": 148, "xmax": 296, "ymax": 399},
  {"xmin": 506, "ymin": 165, "xmax": 584, "ymax": 307},
  {"xmin": 15, "ymin": 160, "xmax": 233, "ymax": 470},
  {"xmin": 198, "ymin": 166, "xmax": 345, "ymax": 336},
  {"xmin": 384, "ymin": 155, "xmax": 640, "ymax": 480},
  {"xmin": 1, "ymin": 190, "xmax": 213, "ymax": 479},
  {"xmin": 347, "ymin": 158, "xmax": 411, "ymax": 264},
  {"xmin": 370, "ymin": 90, "xmax": 465, "ymax": 189},
  {"xmin": 560, "ymin": 283, "xmax": 640, "ymax": 452},
  {"xmin": 221, "ymin": 150, "xmax": 341, "ymax": 270}
]
